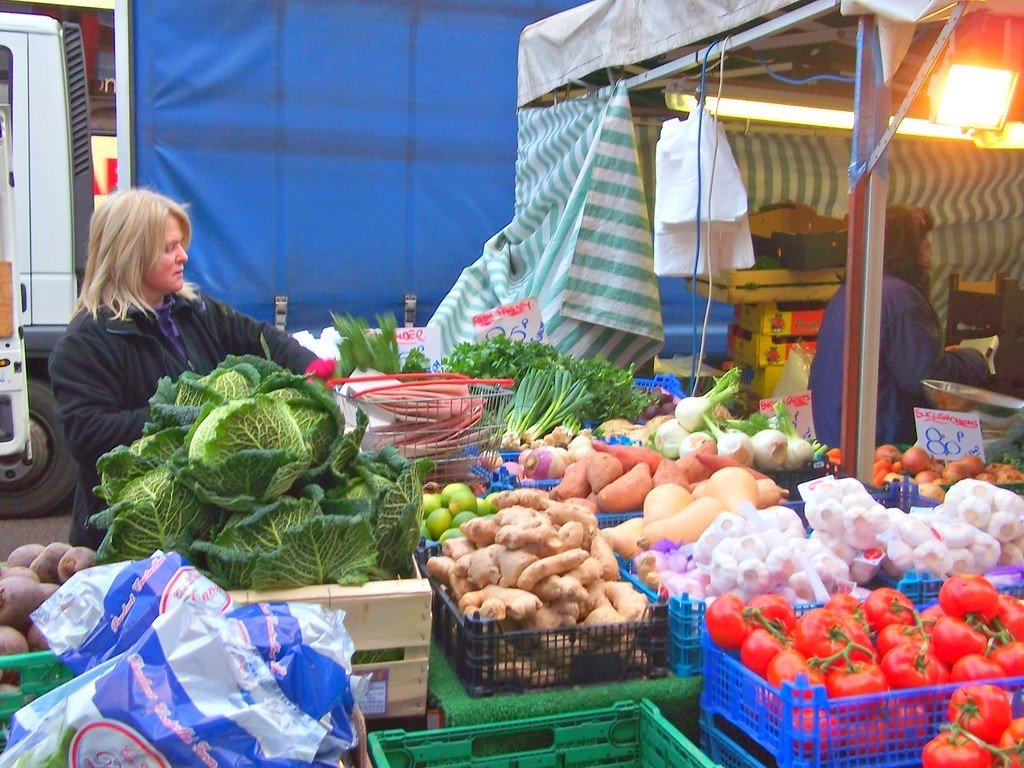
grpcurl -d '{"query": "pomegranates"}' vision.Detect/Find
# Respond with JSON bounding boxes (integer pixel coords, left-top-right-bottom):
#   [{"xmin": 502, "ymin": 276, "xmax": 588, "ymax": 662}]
[{"xmin": 874, "ymin": 445, "xmax": 1023, "ymax": 504}]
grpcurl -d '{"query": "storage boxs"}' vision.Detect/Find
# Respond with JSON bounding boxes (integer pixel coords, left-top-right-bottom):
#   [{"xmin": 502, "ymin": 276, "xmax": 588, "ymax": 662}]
[{"xmin": 105, "ymin": 265, "xmax": 1024, "ymax": 768}]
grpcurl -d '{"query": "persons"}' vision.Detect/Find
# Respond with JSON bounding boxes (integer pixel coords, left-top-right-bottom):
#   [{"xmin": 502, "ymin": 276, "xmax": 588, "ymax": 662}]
[
  {"xmin": 808, "ymin": 204, "xmax": 993, "ymax": 455},
  {"xmin": 48, "ymin": 190, "xmax": 318, "ymax": 555}
]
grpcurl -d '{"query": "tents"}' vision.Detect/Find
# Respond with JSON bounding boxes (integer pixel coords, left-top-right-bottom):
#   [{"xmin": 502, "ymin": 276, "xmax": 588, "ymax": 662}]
[{"xmin": 517, "ymin": 0, "xmax": 1023, "ymax": 378}]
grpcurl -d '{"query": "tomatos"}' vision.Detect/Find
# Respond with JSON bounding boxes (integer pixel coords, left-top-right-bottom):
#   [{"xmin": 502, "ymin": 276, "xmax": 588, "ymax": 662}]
[{"xmin": 704, "ymin": 573, "xmax": 1022, "ymax": 766}]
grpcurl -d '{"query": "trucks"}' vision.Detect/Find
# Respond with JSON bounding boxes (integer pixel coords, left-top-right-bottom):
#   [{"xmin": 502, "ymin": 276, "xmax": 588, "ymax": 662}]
[{"xmin": 0, "ymin": 2, "xmax": 589, "ymax": 518}]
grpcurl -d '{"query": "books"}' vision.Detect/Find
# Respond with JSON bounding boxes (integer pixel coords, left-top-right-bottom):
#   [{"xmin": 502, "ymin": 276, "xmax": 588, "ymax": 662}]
[{"xmin": 958, "ymin": 335, "xmax": 1001, "ymax": 373}]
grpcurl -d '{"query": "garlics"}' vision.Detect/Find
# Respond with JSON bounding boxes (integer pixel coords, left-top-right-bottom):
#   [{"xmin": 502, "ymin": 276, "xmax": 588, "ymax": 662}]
[{"xmin": 655, "ymin": 366, "xmax": 812, "ymax": 473}]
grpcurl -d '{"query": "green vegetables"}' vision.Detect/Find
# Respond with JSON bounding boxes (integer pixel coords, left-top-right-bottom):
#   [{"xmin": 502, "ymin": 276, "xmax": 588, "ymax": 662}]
[{"xmin": 329, "ymin": 311, "xmax": 659, "ymax": 425}]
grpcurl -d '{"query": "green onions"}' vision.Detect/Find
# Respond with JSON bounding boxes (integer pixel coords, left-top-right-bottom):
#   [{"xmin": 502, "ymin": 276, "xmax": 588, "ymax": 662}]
[{"xmin": 485, "ymin": 367, "xmax": 594, "ymax": 451}]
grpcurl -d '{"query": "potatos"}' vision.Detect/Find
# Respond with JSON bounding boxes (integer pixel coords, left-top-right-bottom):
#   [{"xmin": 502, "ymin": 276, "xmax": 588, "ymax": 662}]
[{"xmin": 548, "ymin": 441, "xmax": 771, "ymax": 512}]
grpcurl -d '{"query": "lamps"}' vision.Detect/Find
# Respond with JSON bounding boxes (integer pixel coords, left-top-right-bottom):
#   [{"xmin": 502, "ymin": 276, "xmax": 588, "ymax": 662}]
[{"xmin": 923, "ymin": 61, "xmax": 1020, "ymax": 133}]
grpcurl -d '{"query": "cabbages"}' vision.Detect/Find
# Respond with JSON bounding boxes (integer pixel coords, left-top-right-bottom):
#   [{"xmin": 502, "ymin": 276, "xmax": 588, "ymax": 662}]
[{"xmin": 87, "ymin": 353, "xmax": 436, "ymax": 593}]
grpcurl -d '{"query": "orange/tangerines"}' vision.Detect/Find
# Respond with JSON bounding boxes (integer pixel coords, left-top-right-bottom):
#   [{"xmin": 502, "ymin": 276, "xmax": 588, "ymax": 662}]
[{"xmin": 417, "ymin": 481, "xmax": 500, "ymax": 544}]
